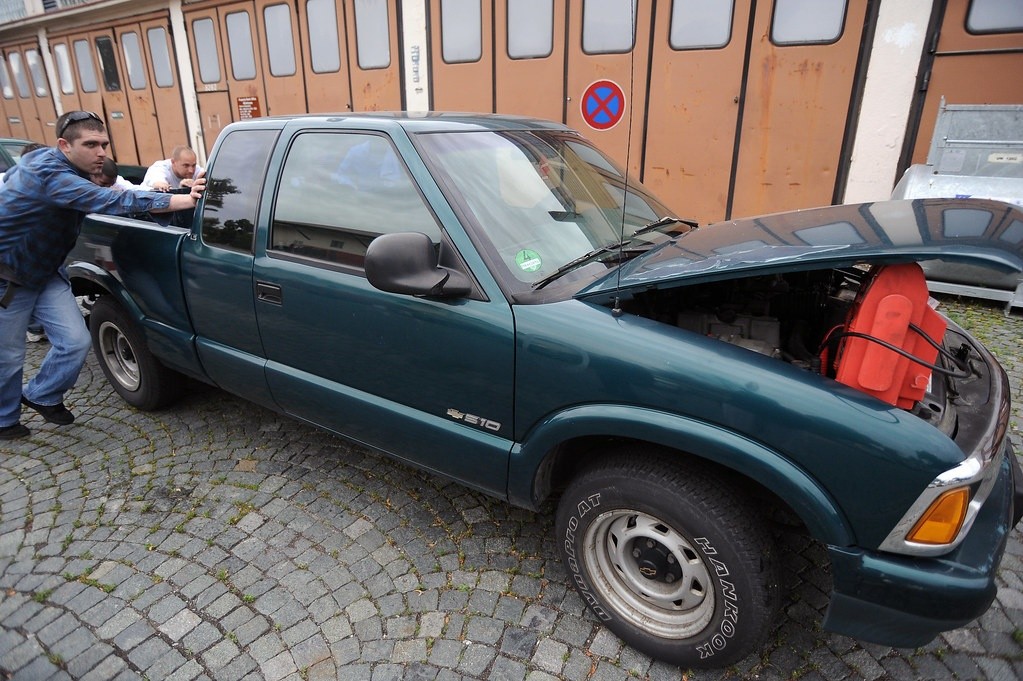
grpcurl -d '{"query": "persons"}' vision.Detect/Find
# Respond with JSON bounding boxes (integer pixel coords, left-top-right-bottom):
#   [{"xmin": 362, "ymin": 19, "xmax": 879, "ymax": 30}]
[
  {"xmin": 335, "ymin": 140, "xmax": 399, "ymax": 191},
  {"xmin": 90, "ymin": 157, "xmax": 153, "ymax": 191},
  {"xmin": 0, "ymin": 111, "xmax": 207, "ymax": 440},
  {"xmin": 141, "ymin": 146, "xmax": 205, "ymax": 192}
]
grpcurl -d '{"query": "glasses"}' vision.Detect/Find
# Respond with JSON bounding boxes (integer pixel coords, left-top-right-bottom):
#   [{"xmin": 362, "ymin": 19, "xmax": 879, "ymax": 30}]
[{"xmin": 59, "ymin": 111, "xmax": 104, "ymax": 137}]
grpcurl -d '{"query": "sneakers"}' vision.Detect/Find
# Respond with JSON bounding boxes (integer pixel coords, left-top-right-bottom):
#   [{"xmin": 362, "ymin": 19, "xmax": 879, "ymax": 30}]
[
  {"xmin": 25, "ymin": 330, "xmax": 48, "ymax": 342},
  {"xmin": 0, "ymin": 423, "xmax": 30, "ymax": 440},
  {"xmin": 21, "ymin": 394, "xmax": 74, "ymax": 425}
]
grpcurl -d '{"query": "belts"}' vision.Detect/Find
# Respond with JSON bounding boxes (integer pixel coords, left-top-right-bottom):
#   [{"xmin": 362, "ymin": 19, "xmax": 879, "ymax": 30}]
[{"xmin": 0, "ymin": 281, "xmax": 14, "ymax": 309}]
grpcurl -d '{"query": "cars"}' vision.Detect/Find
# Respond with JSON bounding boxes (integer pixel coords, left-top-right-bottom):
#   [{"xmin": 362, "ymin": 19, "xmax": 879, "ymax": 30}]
[{"xmin": 0, "ymin": 138, "xmax": 149, "ymax": 185}]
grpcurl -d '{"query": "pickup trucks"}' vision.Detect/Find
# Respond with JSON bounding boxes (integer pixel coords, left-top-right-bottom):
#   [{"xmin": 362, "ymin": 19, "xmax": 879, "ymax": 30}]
[{"xmin": 65, "ymin": 112, "xmax": 1022, "ymax": 673}]
